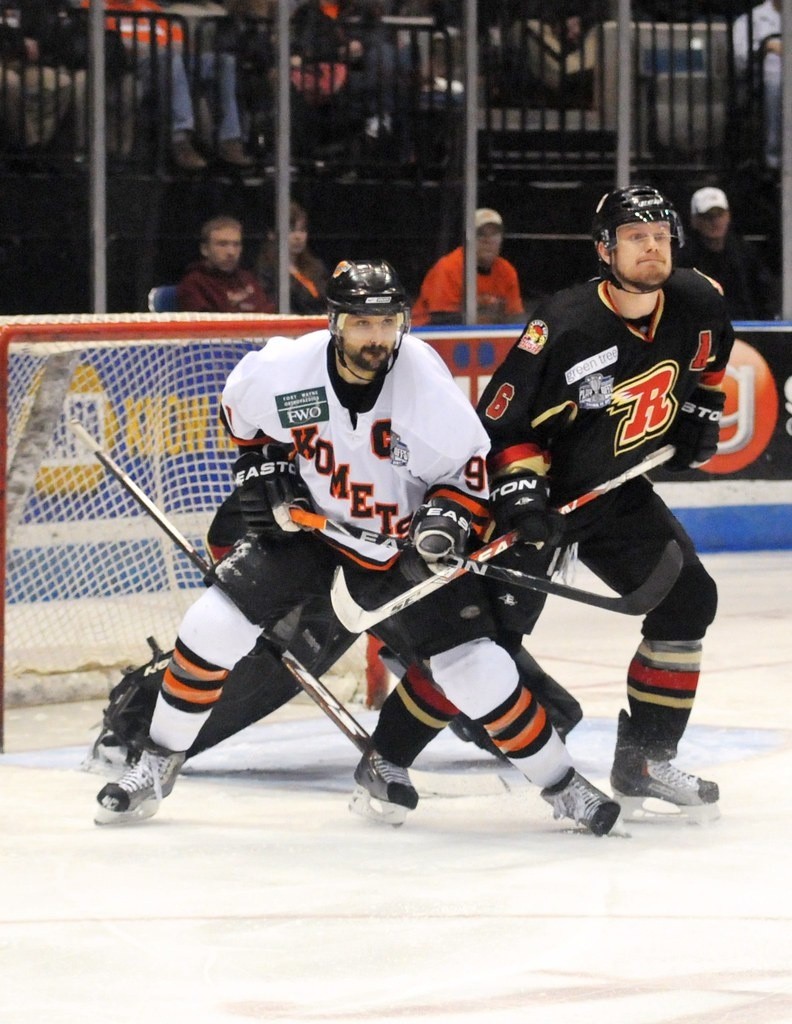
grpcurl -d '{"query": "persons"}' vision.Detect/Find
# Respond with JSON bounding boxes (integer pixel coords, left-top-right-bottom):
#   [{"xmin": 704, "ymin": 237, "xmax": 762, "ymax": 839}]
[
  {"xmin": 683, "ymin": 188, "xmax": 783, "ymax": 321},
  {"xmin": 257, "ymin": 201, "xmax": 327, "ymax": 315},
  {"xmin": 175, "ymin": 216, "xmax": 279, "ymax": 314},
  {"xmin": 98, "ymin": 257, "xmax": 621, "ymax": 837},
  {"xmin": 354, "ymin": 184, "xmax": 719, "ymax": 811},
  {"xmin": 496, "ymin": 0, "xmax": 782, "ymax": 184},
  {"xmin": 0, "ymin": 0, "xmax": 542, "ymax": 166},
  {"xmin": 101, "ymin": 484, "xmax": 584, "ymax": 763},
  {"xmin": 411, "ymin": 207, "xmax": 531, "ymax": 327}
]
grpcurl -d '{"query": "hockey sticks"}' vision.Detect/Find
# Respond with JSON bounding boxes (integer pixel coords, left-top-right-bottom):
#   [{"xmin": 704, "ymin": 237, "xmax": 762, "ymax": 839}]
[
  {"xmin": 327, "ymin": 442, "xmax": 678, "ymax": 634},
  {"xmin": 287, "ymin": 506, "xmax": 686, "ymax": 616},
  {"xmin": 66, "ymin": 414, "xmax": 513, "ymax": 801}
]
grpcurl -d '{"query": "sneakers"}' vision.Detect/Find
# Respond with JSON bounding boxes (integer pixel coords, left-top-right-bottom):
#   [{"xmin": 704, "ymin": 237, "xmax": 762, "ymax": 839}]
[
  {"xmin": 541, "ymin": 766, "xmax": 632, "ymax": 838},
  {"xmin": 610, "ymin": 710, "xmax": 721, "ymax": 824},
  {"xmin": 348, "ymin": 733, "xmax": 418, "ymax": 825},
  {"xmin": 93, "ymin": 743, "xmax": 185, "ymax": 827}
]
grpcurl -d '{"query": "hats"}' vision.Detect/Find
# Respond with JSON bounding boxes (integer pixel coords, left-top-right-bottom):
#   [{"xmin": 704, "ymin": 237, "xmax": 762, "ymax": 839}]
[
  {"xmin": 691, "ymin": 186, "xmax": 729, "ymax": 216},
  {"xmin": 473, "ymin": 209, "xmax": 503, "ymax": 229}
]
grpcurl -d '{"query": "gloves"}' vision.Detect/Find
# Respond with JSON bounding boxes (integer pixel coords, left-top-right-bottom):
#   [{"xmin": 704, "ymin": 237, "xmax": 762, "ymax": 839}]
[
  {"xmin": 491, "ymin": 472, "xmax": 565, "ymax": 546},
  {"xmin": 660, "ymin": 386, "xmax": 726, "ymax": 473},
  {"xmin": 399, "ymin": 496, "xmax": 473, "ymax": 585},
  {"xmin": 233, "ymin": 443, "xmax": 318, "ymax": 538}
]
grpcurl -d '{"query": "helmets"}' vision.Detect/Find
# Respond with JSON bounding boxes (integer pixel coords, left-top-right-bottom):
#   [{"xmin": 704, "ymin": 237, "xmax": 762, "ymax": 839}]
[
  {"xmin": 591, "ymin": 186, "xmax": 686, "ymax": 263},
  {"xmin": 326, "ymin": 259, "xmax": 410, "ymax": 338}
]
